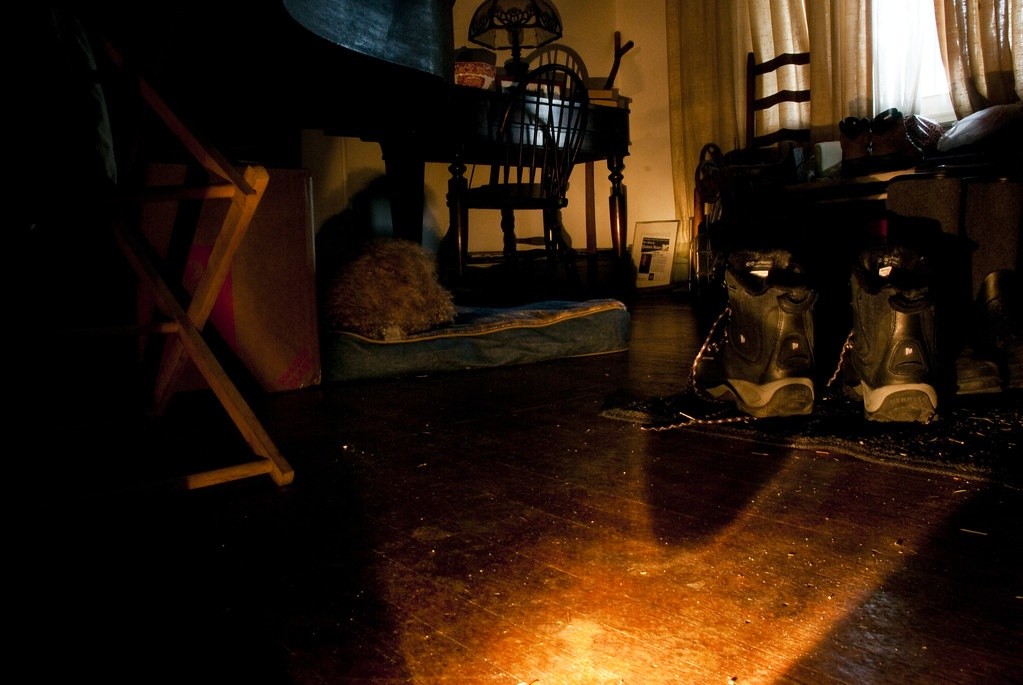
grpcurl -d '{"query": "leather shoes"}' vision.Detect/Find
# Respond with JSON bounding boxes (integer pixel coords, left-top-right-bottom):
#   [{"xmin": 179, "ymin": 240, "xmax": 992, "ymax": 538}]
[
  {"xmin": 873, "ymin": 110, "xmax": 923, "ymax": 170},
  {"xmin": 956, "ymin": 349, "xmax": 998, "ymax": 395},
  {"xmin": 839, "ymin": 117, "xmax": 871, "ymax": 176},
  {"xmin": 1008, "ymin": 345, "xmax": 1022, "ymax": 388}
]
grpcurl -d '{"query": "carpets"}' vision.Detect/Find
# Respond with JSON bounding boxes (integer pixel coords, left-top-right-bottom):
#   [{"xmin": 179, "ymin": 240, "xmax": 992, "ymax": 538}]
[{"xmin": 599, "ymin": 388, "xmax": 1023, "ymax": 492}]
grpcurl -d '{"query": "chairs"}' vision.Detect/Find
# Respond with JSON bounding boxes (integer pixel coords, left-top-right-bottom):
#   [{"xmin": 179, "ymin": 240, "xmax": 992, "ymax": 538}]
[
  {"xmin": 745, "ymin": 52, "xmax": 812, "ymax": 147},
  {"xmin": 457, "ymin": 64, "xmax": 590, "ymax": 277},
  {"xmin": 102, "ymin": 37, "xmax": 294, "ymax": 490}
]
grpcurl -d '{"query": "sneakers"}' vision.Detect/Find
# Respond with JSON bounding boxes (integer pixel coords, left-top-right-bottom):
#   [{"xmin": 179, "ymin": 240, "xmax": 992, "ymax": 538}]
[
  {"xmin": 691, "ymin": 248, "xmax": 820, "ymax": 420},
  {"xmin": 839, "ymin": 247, "xmax": 940, "ymax": 427}
]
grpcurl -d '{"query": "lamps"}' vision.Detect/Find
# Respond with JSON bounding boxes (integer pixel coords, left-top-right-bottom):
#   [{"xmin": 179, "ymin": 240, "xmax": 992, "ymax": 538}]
[{"xmin": 469, "ymin": 0, "xmax": 562, "ymax": 76}]
